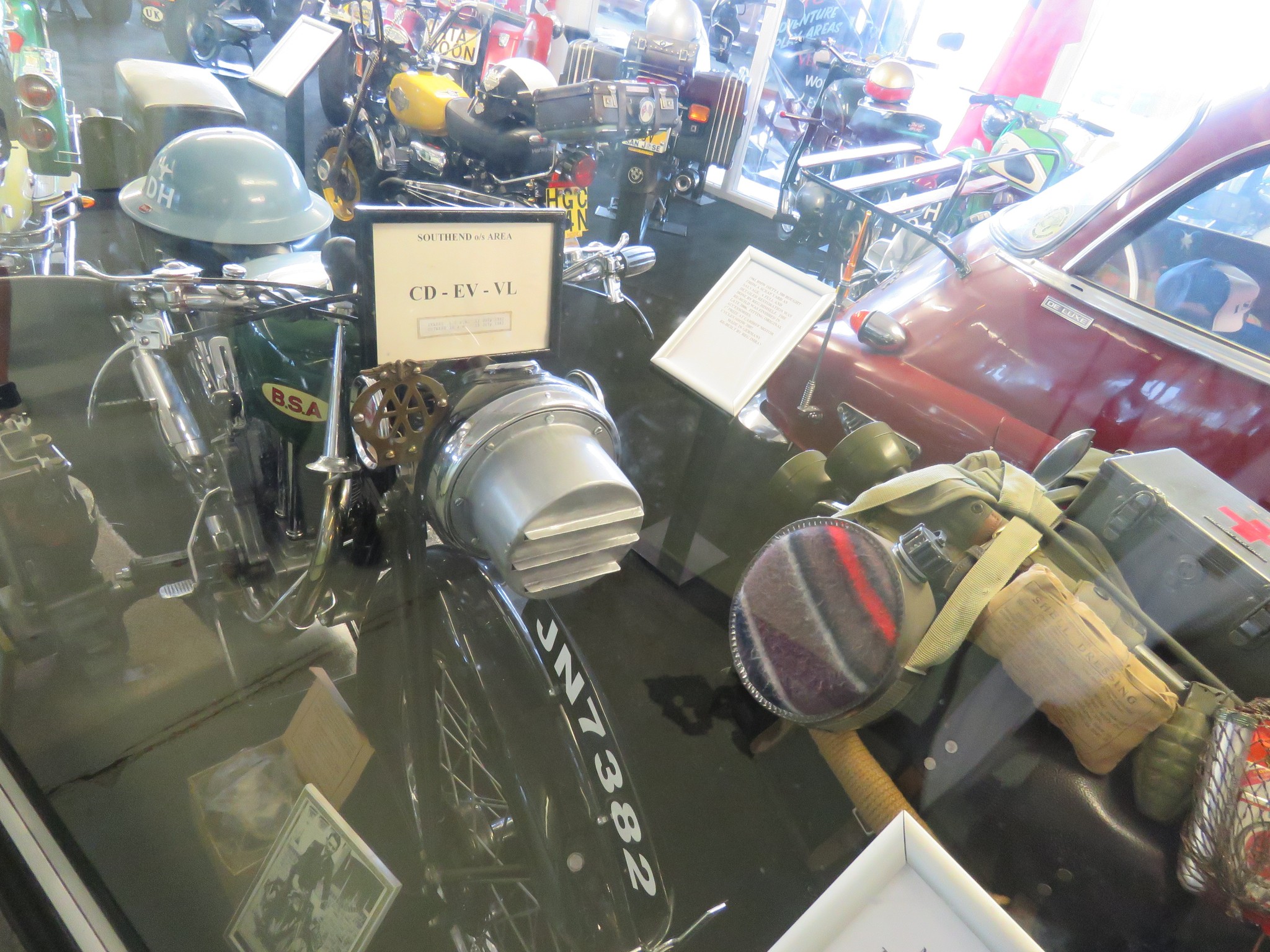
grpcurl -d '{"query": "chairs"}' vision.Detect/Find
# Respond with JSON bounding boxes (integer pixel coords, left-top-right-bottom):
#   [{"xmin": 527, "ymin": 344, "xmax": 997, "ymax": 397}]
[{"xmin": 1153, "ymin": 257, "xmax": 1260, "ymax": 336}]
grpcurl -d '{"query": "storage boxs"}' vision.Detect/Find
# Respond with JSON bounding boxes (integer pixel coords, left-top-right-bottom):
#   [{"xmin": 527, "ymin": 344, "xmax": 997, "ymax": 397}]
[
  {"xmin": 184, "ymin": 663, "xmax": 375, "ymax": 906},
  {"xmin": 113, "ymin": 57, "xmax": 249, "ymax": 179},
  {"xmin": 1064, "ymin": 445, "xmax": 1270, "ymax": 702},
  {"xmin": 626, "ymin": 30, "xmax": 701, "ymax": 79},
  {"xmin": 531, "ymin": 77, "xmax": 679, "ymax": 146},
  {"xmin": 559, "ymin": 38, "xmax": 624, "ymax": 86}
]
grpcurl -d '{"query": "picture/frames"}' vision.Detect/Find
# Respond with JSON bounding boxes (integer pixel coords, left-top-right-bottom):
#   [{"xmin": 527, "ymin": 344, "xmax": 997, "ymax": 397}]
[
  {"xmin": 245, "ymin": 13, "xmax": 344, "ymax": 105},
  {"xmin": 649, "ymin": 246, "xmax": 839, "ymax": 427},
  {"xmin": 218, "ymin": 781, "xmax": 402, "ymax": 952},
  {"xmin": 353, "ymin": 204, "xmax": 566, "ymax": 375},
  {"xmin": 754, "ymin": 806, "xmax": 1048, "ymax": 952}
]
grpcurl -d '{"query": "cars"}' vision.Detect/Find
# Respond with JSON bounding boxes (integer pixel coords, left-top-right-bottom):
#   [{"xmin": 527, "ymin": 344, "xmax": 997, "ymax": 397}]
[{"xmin": 767, "ymin": 82, "xmax": 1270, "ymax": 567}]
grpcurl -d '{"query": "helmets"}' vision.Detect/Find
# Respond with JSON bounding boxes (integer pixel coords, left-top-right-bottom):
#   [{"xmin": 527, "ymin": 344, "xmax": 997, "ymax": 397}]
[
  {"xmin": 862, "ymin": 61, "xmax": 914, "ymax": 105},
  {"xmin": 467, "ymin": 56, "xmax": 558, "ymax": 122},
  {"xmin": 118, "ymin": 128, "xmax": 334, "ymax": 244}
]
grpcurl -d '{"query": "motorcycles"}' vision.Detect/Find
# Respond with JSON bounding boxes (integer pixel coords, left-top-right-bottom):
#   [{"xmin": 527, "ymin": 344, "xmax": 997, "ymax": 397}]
[{"xmin": 81, "ymin": 6, "xmax": 1118, "ymax": 952}]
